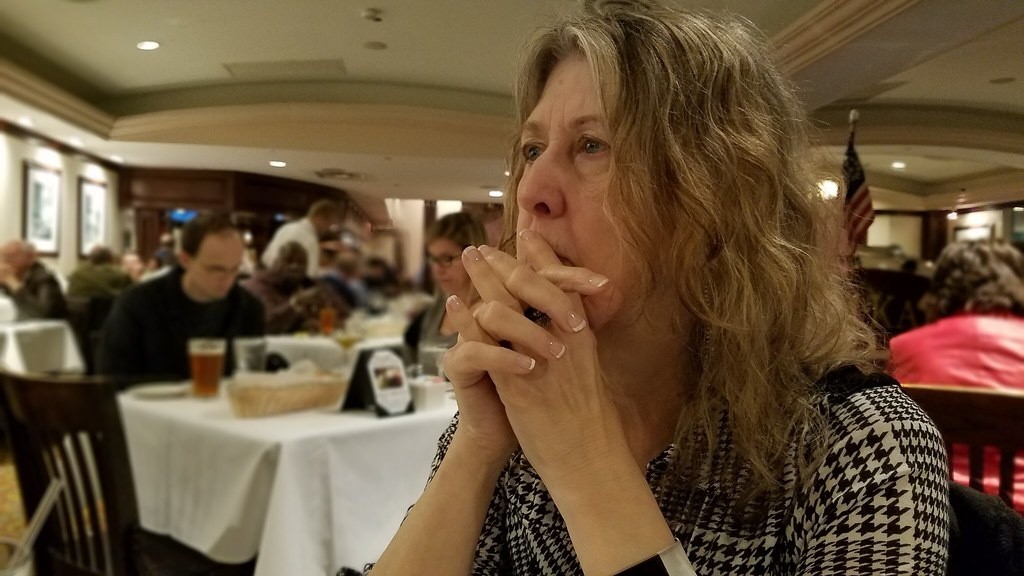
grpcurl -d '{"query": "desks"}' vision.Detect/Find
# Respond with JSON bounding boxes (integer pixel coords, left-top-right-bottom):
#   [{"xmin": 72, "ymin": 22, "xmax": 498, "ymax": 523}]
[
  {"xmin": 0, "ymin": 319, "xmax": 87, "ymax": 377},
  {"xmin": 112, "ymin": 377, "xmax": 458, "ymax": 576}
]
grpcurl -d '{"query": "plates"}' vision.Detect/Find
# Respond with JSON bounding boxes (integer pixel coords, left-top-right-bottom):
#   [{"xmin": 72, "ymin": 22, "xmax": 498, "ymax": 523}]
[{"xmin": 446, "ymin": 382, "xmax": 454, "ymax": 391}]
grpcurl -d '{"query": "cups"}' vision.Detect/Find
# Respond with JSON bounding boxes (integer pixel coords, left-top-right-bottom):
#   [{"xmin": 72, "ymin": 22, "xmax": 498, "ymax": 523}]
[
  {"xmin": 233, "ymin": 338, "xmax": 268, "ymax": 375},
  {"xmin": 408, "ymin": 364, "xmax": 446, "ymax": 412},
  {"xmin": 188, "ymin": 339, "xmax": 226, "ymax": 396}
]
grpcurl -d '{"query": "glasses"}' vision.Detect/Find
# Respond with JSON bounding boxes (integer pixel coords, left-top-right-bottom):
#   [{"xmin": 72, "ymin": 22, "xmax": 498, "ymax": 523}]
[
  {"xmin": 192, "ymin": 258, "xmax": 239, "ymax": 276},
  {"xmin": 426, "ymin": 252, "xmax": 464, "ymax": 266}
]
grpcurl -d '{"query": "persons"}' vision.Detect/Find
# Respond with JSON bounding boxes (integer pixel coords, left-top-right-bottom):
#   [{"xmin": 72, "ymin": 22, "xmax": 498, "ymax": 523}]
[
  {"xmin": 101, "ymin": 208, "xmax": 266, "ymax": 447},
  {"xmin": 337, "ymin": 3, "xmax": 950, "ymax": 576},
  {"xmin": 0, "ymin": 196, "xmax": 1023, "ymax": 507}
]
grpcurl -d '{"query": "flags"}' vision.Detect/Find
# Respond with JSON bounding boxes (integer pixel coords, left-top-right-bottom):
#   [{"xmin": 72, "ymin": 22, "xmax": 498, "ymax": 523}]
[{"xmin": 842, "ymin": 147, "xmax": 875, "ymax": 256}]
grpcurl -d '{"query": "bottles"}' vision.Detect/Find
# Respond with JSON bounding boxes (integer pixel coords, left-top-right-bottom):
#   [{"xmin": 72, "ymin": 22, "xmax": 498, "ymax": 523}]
[{"xmin": 321, "ymin": 300, "xmax": 335, "ymax": 338}]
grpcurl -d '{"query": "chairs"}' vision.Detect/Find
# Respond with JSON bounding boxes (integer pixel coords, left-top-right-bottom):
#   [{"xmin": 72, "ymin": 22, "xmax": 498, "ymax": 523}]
[
  {"xmin": 0, "ymin": 369, "xmax": 257, "ymax": 576},
  {"xmin": 900, "ymin": 384, "xmax": 1024, "ymax": 514}
]
